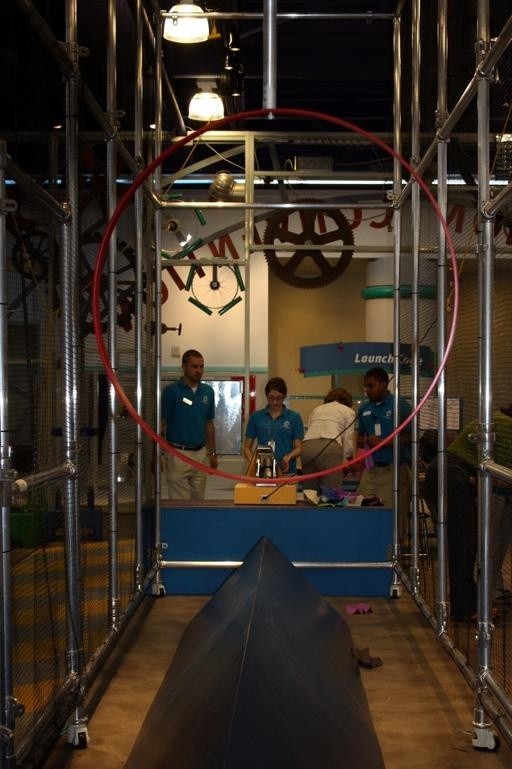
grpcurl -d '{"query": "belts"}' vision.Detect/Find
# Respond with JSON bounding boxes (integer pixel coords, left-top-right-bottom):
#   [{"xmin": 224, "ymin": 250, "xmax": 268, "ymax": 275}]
[
  {"xmin": 372, "ymin": 463, "xmax": 393, "ymax": 467},
  {"xmin": 167, "ymin": 444, "xmax": 206, "ymax": 451}
]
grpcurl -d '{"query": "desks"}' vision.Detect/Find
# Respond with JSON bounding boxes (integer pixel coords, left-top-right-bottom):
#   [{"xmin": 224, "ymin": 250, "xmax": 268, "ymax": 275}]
[{"xmin": 159, "ymin": 499, "xmax": 394, "ymax": 597}]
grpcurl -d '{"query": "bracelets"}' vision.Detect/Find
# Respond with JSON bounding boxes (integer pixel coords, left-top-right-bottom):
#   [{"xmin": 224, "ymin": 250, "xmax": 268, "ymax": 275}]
[
  {"xmin": 208, "ymin": 451, "xmax": 216, "ymax": 457},
  {"xmin": 287, "ymin": 455, "xmax": 290, "ymax": 462}
]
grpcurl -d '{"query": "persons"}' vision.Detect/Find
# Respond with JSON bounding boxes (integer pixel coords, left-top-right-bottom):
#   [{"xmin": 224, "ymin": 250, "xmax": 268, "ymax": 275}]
[
  {"xmin": 301, "ymin": 388, "xmax": 357, "ymax": 494},
  {"xmin": 160, "ymin": 350, "xmax": 218, "ymax": 499},
  {"xmin": 244, "ymin": 377, "xmax": 304, "ymax": 473},
  {"xmin": 422, "ymin": 407, "xmax": 512, "ymax": 622},
  {"xmin": 492, "ymin": 478, "xmax": 512, "ymax": 605},
  {"xmin": 356, "ymin": 367, "xmax": 411, "ymax": 542}
]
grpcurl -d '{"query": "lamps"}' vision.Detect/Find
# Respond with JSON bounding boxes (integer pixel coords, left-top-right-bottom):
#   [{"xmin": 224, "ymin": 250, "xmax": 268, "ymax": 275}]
[
  {"xmin": 163, "ymin": 0, "xmax": 209, "ymax": 44},
  {"xmin": 187, "ymin": 80, "xmax": 226, "ymax": 120}
]
